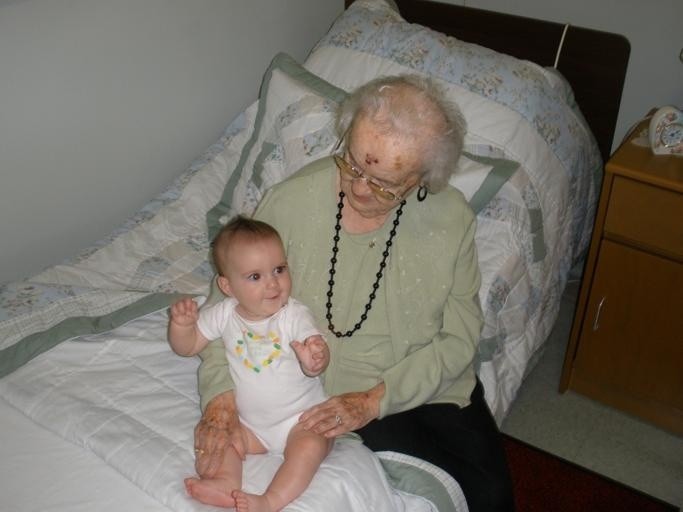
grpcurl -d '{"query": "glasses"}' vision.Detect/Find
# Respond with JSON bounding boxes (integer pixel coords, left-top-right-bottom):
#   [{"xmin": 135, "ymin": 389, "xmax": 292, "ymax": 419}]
[{"xmin": 333, "ymin": 124, "xmax": 426, "ymax": 201}]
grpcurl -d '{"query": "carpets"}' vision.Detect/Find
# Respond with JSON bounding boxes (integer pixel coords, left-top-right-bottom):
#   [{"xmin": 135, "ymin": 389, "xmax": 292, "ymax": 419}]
[{"xmin": 496, "ymin": 433, "xmax": 682, "ymax": 512}]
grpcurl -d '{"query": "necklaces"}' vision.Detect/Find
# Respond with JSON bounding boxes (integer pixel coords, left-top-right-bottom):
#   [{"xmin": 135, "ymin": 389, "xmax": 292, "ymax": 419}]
[{"xmin": 325, "ymin": 192, "xmax": 408, "ymax": 338}]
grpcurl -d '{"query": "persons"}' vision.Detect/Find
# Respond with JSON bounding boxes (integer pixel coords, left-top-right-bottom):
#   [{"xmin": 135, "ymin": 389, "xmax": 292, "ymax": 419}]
[
  {"xmin": 167, "ymin": 215, "xmax": 335, "ymax": 511},
  {"xmin": 193, "ymin": 73, "xmax": 515, "ymax": 511}
]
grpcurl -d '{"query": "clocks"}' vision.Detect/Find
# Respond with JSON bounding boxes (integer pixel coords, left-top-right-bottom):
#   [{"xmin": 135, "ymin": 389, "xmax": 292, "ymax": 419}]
[{"xmin": 648, "ymin": 107, "xmax": 683, "ymax": 157}]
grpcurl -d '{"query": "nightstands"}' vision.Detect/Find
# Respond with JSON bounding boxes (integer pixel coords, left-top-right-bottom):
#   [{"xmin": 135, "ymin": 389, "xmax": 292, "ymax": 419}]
[{"xmin": 554, "ymin": 106, "xmax": 683, "ymax": 442}]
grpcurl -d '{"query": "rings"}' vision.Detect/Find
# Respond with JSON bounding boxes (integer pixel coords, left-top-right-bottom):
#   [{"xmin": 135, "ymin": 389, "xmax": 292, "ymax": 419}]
[
  {"xmin": 199, "ymin": 447, "xmax": 206, "ymax": 453},
  {"xmin": 194, "ymin": 447, "xmax": 200, "ymax": 453},
  {"xmin": 336, "ymin": 415, "xmax": 341, "ymax": 424}
]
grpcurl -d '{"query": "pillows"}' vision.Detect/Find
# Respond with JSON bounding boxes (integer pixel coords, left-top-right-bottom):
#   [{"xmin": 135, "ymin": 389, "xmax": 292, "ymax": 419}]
[{"xmin": 203, "ymin": 49, "xmax": 520, "ymax": 266}]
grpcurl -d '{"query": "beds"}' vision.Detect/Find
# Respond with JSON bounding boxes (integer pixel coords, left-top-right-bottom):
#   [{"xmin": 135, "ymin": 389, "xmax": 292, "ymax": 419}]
[{"xmin": 1, "ymin": 0, "xmax": 634, "ymax": 512}]
